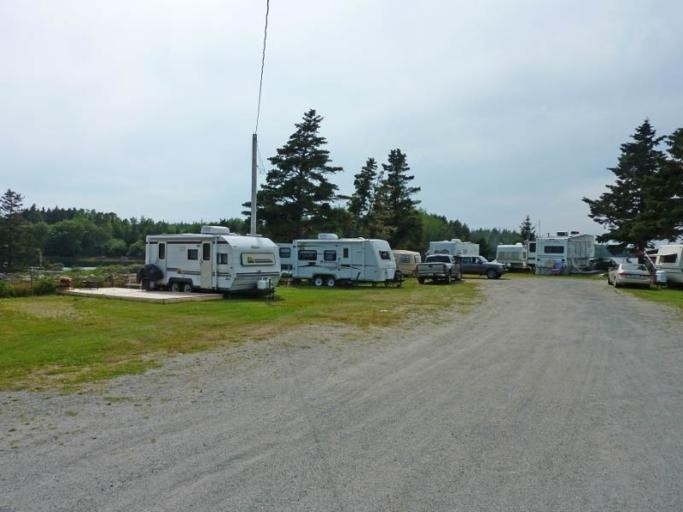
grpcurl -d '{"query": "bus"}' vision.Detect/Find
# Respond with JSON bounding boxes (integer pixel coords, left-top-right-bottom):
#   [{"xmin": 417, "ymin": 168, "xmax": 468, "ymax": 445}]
[{"xmin": 391, "ymin": 250, "xmax": 421, "ymax": 277}]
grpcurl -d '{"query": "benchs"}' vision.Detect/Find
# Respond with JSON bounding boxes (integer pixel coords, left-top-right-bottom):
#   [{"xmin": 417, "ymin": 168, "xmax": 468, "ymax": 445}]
[{"xmin": 511, "ymin": 266, "xmax": 532, "ymax": 274}]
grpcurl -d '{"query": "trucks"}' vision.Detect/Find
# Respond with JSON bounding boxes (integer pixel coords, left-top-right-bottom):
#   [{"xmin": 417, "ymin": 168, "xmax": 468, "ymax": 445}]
[
  {"xmin": 535, "ymin": 233, "xmax": 595, "ymax": 271},
  {"xmin": 494, "ymin": 244, "xmax": 526, "ymax": 271},
  {"xmin": 424, "ymin": 239, "xmax": 480, "ymax": 257},
  {"xmin": 643, "ymin": 244, "xmax": 683, "ymax": 285},
  {"xmin": 525, "ymin": 241, "xmax": 535, "ymax": 266}
]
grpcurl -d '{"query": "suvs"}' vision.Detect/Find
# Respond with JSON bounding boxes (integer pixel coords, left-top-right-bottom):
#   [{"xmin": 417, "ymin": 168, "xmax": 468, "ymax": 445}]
[{"xmin": 453, "ymin": 255, "xmax": 509, "ymax": 279}]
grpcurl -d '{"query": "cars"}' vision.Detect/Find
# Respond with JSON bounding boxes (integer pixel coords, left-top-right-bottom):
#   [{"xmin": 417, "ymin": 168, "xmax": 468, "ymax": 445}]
[{"xmin": 608, "ymin": 263, "xmax": 651, "ymax": 289}]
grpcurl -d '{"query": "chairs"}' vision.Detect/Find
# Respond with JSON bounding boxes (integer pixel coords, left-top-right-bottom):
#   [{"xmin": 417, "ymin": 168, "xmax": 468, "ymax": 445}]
[{"xmin": 124, "ymin": 273, "xmax": 144, "ymax": 292}]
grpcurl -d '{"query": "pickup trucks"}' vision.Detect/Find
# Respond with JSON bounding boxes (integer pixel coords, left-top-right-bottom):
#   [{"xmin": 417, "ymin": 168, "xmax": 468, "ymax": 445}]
[{"xmin": 416, "ymin": 253, "xmax": 461, "ymax": 285}]
